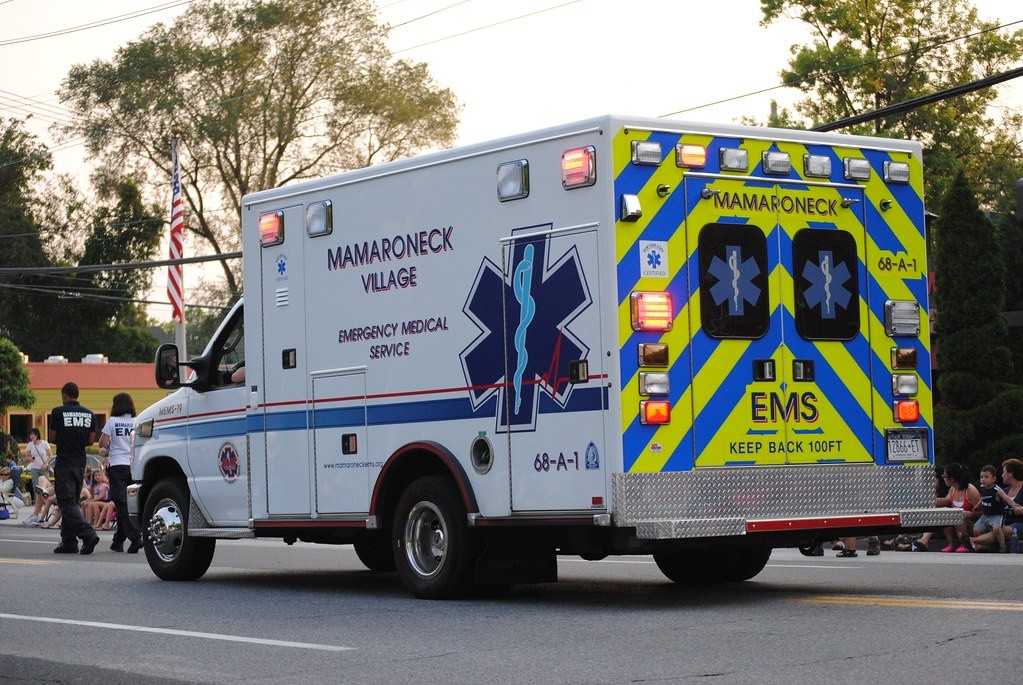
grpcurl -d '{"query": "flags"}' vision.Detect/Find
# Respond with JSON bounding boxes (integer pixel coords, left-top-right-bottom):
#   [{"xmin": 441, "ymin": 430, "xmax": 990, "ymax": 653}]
[{"xmin": 167, "ymin": 147, "xmax": 184, "ymax": 324}]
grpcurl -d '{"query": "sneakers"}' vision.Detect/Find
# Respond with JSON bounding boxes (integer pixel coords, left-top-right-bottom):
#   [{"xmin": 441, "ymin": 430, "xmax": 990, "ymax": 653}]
[
  {"xmin": 110, "ymin": 542, "xmax": 124, "ymax": 552},
  {"xmin": 80, "ymin": 534, "xmax": 99, "ymax": 554},
  {"xmin": 22, "ymin": 514, "xmax": 37, "ymax": 524},
  {"xmin": 34, "ymin": 485, "xmax": 49, "ymax": 498},
  {"xmin": 54, "ymin": 542, "xmax": 79, "ymax": 552},
  {"xmin": 867, "ymin": 537, "xmax": 880, "ymax": 555},
  {"xmin": 128, "ymin": 538, "xmax": 143, "ymax": 553}
]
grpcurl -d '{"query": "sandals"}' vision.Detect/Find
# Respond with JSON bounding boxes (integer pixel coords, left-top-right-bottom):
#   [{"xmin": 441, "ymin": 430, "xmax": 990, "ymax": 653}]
[{"xmin": 836, "ymin": 548, "xmax": 858, "ymax": 557}]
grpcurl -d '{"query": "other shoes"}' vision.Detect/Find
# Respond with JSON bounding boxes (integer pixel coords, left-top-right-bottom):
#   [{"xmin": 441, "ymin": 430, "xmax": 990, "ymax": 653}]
[
  {"xmin": 92, "ymin": 524, "xmax": 110, "ymax": 531},
  {"xmin": 961, "ymin": 533, "xmax": 976, "ymax": 552},
  {"xmin": 24, "ymin": 496, "xmax": 29, "ymax": 506},
  {"xmin": 941, "ymin": 545, "xmax": 955, "ymax": 552},
  {"xmin": 956, "ymin": 546, "xmax": 969, "ymax": 553},
  {"xmin": 41, "ymin": 522, "xmax": 61, "ymax": 529},
  {"xmin": 999, "ymin": 546, "xmax": 1007, "ymax": 552},
  {"xmin": 913, "ymin": 538, "xmax": 928, "ymax": 551},
  {"xmin": 32, "ymin": 519, "xmax": 44, "ymax": 524},
  {"xmin": 832, "ymin": 541, "xmax": 845, "ymax": 550}
]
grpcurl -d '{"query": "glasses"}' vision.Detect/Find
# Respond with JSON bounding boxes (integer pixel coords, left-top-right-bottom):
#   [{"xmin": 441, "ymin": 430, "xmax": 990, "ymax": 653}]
[
  {"xmin": 942, "ymin": 475, "xmax": 948, "ymax": 480},
  {"xmin": 0, "ymin": 474, "xmax": 9, "ymax": 476}
]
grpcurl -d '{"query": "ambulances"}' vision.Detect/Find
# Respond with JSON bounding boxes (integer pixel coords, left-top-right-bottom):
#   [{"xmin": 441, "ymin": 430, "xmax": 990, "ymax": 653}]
[{"xmin": 125, "ymin": 111, "xmax": 965, "ymax": 603}]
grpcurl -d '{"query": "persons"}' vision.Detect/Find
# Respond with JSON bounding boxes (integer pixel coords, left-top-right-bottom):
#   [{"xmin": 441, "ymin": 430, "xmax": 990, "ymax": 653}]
[
  {"xmin": 99, "ymin": 393, "xmax": 144, "ymax": 554},
  {"xmin": 232, "ymin": 366, "xmax": 245, "ymax": 384},
  {"xmin": 162, "ymin": 355, "xmax": 177, "ymax": 385},
  {"xmin": 0, "ymin": 427, "xmax": 120, "ymax": 532},
  {"xmin": 48, "ymin": 382, "xmax": 99, "ymax": 554},
  {"xmin": 808, "ymin": 458, "xmax": 1023, "ymax": 558}
]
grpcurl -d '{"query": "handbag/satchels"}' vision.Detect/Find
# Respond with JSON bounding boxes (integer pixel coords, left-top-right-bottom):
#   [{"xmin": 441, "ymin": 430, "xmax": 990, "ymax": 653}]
[
  {"xmin": 0, "ymin": 509, "xmax": 10, "ymax": 520},
  {"xmin": 47, "ymin": 463, "xmax": 54, "ymax": 477}
]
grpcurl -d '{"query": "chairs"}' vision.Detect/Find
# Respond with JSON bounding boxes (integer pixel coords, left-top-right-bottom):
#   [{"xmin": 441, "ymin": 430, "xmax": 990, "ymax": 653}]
[{"xmin": 0, "ymin": 465, "xmax": 24, "ymax": 518}]
